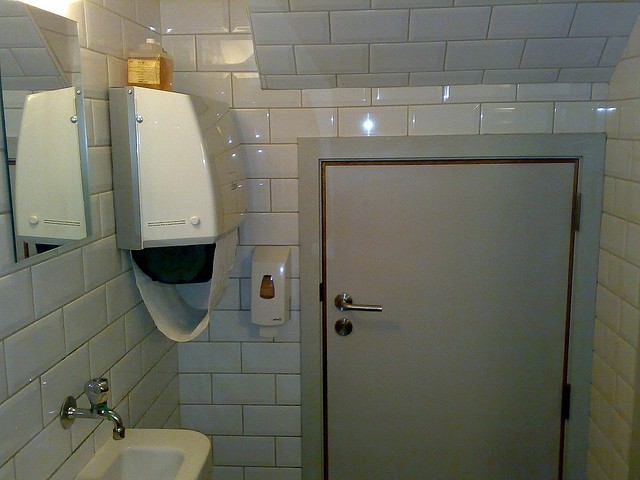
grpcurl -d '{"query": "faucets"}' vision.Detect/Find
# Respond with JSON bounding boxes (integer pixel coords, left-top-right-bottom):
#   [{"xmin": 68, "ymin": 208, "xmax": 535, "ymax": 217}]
[{"xmin": 61, "ymin": 378, "xmax": 125, "ymax": 441}]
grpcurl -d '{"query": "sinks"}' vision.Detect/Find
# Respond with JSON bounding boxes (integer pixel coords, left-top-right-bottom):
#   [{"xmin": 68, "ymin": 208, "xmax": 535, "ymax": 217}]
[{"xmin": 74, "ymin": 427, "xmax": 212, "ymax": 480}]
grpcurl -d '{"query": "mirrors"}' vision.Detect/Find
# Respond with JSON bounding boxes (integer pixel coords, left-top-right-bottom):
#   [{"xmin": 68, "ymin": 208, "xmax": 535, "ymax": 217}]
[{"xmin": 0, "ymin": 0, "xmax": 94, "ymax": 279}]
